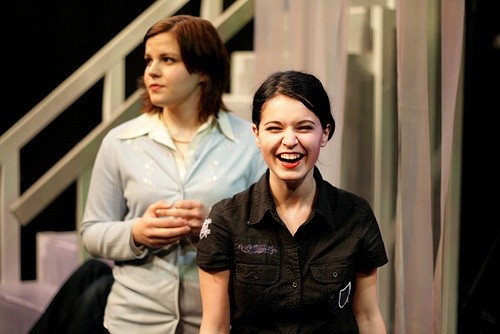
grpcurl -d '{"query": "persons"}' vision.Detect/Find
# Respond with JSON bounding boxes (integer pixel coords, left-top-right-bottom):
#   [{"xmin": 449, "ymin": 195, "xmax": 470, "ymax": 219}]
[
  {"xmin": 196, "ymin": 70, "xmax": 390, "ymax": 334},
  {"xmin": 79, "ymin": 14, "xmax": 270, "ymax": 334}
]
